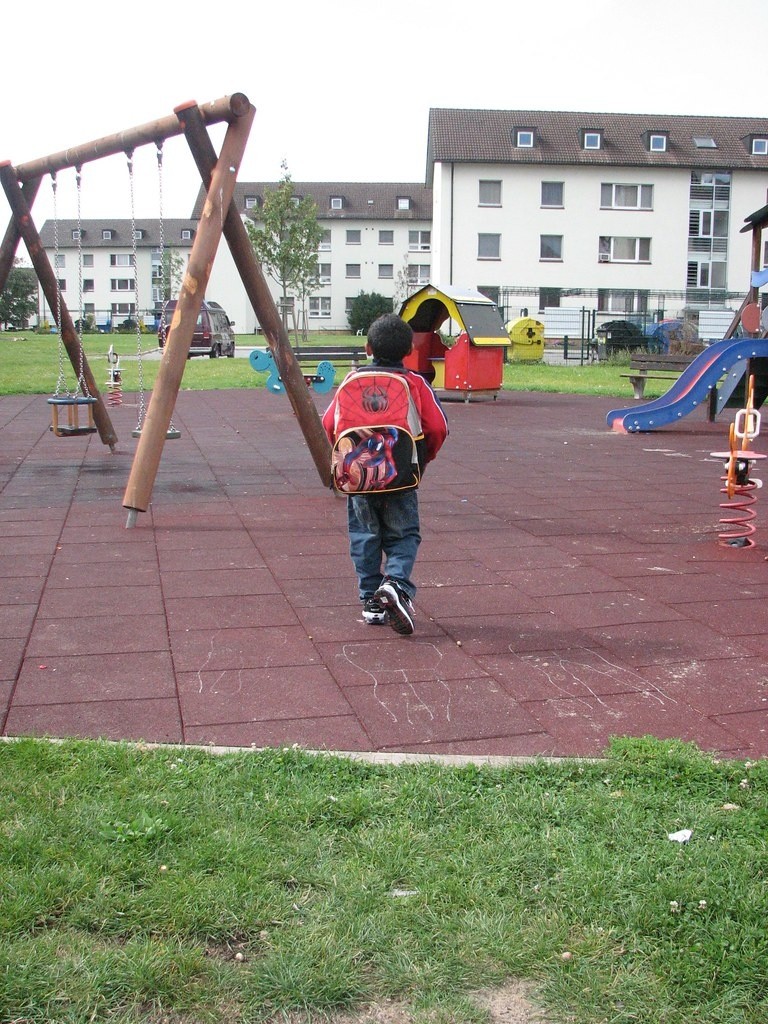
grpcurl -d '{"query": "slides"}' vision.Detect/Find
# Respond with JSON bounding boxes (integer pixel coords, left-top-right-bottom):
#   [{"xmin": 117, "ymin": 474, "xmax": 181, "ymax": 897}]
[{"xmin": 606, "ymin": 339, "xmax": 768, "ymax": 434}]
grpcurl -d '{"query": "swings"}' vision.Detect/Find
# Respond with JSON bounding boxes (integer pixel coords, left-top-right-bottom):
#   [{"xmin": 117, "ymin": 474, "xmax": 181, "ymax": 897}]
[
  {"xmin": 47, "ymin": 169, "xmax": 98, "ymax": 437},
  {"xmin": 126, "ymin": 139, "xmax": 181, "ymax": 441}
]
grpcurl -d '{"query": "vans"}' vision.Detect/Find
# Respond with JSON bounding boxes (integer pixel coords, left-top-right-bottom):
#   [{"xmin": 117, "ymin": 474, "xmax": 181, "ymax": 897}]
[{"xmin": 157, "ymin": 300, "xmax": 237, "ymax": 359}]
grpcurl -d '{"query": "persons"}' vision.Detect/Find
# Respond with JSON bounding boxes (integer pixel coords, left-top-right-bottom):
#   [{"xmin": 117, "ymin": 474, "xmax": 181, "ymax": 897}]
[{"xmin": 321, "ymin": 315, "xmax": 449, "ymax": 636}]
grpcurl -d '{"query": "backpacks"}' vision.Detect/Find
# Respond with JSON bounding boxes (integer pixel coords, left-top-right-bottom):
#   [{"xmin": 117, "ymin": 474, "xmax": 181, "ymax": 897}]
[{"xmin": 330, "ymin": 371, "xmax": 426, "ymax": 498}]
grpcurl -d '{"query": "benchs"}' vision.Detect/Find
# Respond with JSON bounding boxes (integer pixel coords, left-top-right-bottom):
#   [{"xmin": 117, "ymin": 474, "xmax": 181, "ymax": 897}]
[
  {"xmin": 265, "ymin": 346, "xmax": 367, "ymax": 373},
  {"xmin": 620, "ymin": 354, "xmax": 697, "ymax": 400}
]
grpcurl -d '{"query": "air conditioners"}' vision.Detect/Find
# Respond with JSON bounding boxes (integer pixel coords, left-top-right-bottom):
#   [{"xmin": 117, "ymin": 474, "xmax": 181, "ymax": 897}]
[{"xmin": 600, "ymin": 253, "xmax": 611, "ymax": 261}]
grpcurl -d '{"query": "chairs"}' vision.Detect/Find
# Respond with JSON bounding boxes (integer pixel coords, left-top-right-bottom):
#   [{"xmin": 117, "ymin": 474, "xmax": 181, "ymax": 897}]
[{"xmin": 356, "ymin": 328, "xmax": 365, "ymax": 336}]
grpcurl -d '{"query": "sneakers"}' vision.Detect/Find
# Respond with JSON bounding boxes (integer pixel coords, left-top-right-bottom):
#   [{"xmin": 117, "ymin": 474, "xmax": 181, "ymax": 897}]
[
  {"xmin": 361, "ymin": 598, "xmax": 386, "ymax": 624},
  {"xmin": 373, "ymin": 580, "xmax": 415, "ymax": 634}
]
